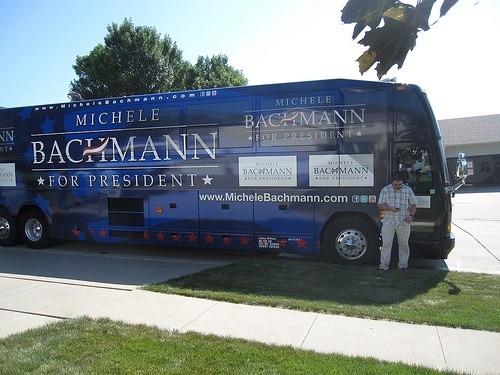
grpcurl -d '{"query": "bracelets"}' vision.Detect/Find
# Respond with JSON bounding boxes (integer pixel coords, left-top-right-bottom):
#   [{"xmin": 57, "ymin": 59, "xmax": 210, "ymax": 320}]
[{"xmin": 409, "ymin": 213, "xmax": 413, "ymax": 217}]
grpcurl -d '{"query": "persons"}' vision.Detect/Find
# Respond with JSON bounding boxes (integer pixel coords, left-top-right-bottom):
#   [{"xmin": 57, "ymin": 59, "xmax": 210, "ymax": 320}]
[{"xmin": 377, "ymin": 172, "xmax": 417, "ymax": 273}]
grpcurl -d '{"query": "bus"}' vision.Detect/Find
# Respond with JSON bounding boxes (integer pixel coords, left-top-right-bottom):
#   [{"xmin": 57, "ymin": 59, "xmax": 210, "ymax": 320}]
[{"xmin": 0, "ymin": 78, "xmax": 468, "ymax": 268}]
